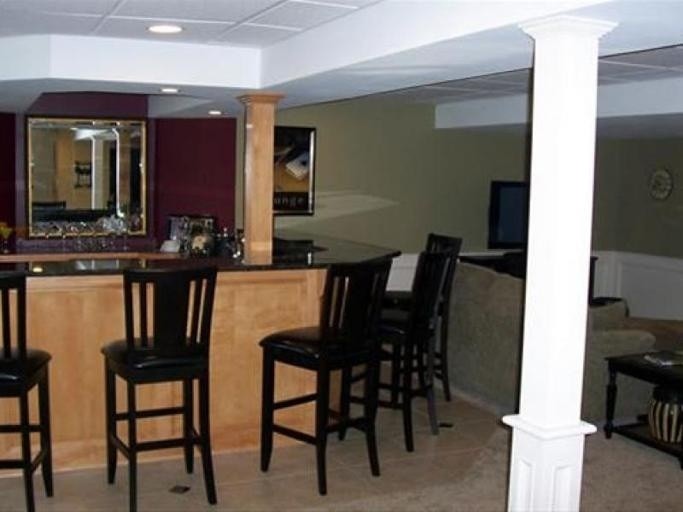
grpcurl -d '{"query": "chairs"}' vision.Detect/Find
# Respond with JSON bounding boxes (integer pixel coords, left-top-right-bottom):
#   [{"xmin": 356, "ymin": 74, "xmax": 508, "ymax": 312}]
[
  {"xmin": 352, "ymin": 248, "xmax": 451, "ymax": 451},
  {"xmin": 101, "ymin": 264, "xmax": 219, "ymax": 510},
  {"xmin": 258, "ymin": 261, "xmax": 382, "ymax": 495},
  {"xmin": 0, "ymin": 270, "xmax": 53, "ymax": 510},
  {"xmin": 383, "ymin": 233, "xmax": 463, "ymax": 400}
]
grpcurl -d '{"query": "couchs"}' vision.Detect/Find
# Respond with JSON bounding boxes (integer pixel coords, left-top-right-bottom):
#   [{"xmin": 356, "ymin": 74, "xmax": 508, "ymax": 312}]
[{"xmin": 436, "ymin": 262, "xmax": 662, "ymax": 420}]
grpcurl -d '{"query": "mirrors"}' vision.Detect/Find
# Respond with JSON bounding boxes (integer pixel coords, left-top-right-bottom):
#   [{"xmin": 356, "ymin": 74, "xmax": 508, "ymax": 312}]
[{"xmin": 26, "ymin": 117, "xmax": 149, "ymax": 240}]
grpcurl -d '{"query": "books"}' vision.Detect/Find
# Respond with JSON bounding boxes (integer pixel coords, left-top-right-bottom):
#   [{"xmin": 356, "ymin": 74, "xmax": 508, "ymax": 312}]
[{"xmin": 644, "ymin": 349, "xmax": 683, "ymax": 366}]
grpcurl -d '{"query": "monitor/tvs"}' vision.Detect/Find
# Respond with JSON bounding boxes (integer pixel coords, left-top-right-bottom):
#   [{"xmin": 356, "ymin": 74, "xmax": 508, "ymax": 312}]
[{"xmin": 488, "ymin": 179, "xmax": 530, "ymax": 249}]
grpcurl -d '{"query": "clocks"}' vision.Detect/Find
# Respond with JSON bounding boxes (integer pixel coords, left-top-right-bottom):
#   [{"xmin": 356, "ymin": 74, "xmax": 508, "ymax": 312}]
[{"xmin": 648, "ymin": 167, "xmax": 673, "ymax": 200}]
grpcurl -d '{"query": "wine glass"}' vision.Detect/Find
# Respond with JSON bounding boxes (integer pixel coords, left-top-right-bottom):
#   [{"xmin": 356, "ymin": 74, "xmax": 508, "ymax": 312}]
[{"xmin": 32, "ymin": 215, "xmax": 132, "ymax": 252}]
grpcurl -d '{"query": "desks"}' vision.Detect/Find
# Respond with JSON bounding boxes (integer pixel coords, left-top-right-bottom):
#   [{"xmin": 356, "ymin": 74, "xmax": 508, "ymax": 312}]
[{"xmin": 604, "ymin": 346, "xmax": 683, "ymax": 470}]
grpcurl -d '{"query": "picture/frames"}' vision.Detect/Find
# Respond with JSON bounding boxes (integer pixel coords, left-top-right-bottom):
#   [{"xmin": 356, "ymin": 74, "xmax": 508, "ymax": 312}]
[{"xmin": 272, "ymin": 124, "xmax": 315, "ymax": 215}]
